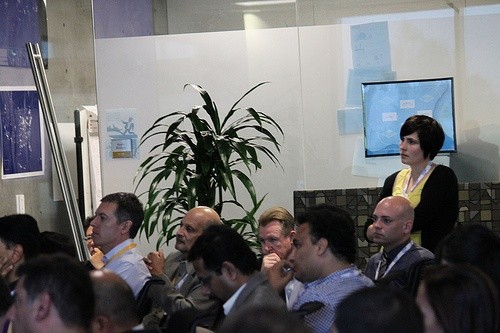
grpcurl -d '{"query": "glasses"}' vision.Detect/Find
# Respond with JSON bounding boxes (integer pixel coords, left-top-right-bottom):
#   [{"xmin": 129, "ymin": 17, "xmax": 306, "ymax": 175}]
[{"xmin": 83, "ymin": 236, "xmax": 92, "ymax": 242}]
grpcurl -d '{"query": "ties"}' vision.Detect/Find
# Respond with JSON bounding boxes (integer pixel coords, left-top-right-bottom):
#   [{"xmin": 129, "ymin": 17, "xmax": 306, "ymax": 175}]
[{"xmin": 377, "ymin": 252, "xmax": 387, "ymax": 279}]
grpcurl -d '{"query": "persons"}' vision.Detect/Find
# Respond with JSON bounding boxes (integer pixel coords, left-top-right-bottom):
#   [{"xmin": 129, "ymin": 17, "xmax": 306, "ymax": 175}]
[
  {"xmin": 364, "ymin": 115, "xmax": 459, "ymax": 254},
  {"xmin": 0, "ymin": 193, "xmax": 500, "ymax": 333}
]
